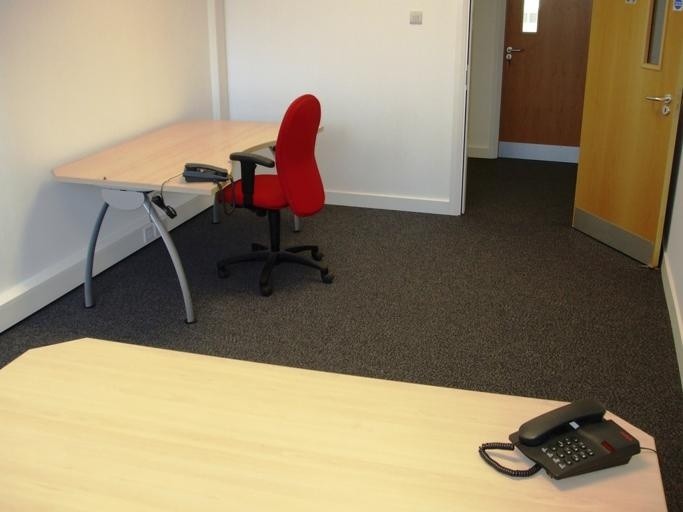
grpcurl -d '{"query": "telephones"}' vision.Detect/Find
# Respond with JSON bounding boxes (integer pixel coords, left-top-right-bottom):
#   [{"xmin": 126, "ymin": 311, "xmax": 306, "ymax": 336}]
[
  {"xmin": 507, "ymin": 399, "xmax": 641, "ymax": 480},
  {"xmin": 183, "ymin": 163, "xmax": 229, "ymax": 183}
]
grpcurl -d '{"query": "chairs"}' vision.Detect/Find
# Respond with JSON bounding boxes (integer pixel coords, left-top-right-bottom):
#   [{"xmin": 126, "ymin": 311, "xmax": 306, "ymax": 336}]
[{"xmin": 215, "ymin": 94, "xmax": 336, "ymax": 297}]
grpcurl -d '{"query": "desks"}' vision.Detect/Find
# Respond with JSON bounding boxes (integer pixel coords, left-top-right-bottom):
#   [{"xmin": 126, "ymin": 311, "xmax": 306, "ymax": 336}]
[
  {"xmin": 0, "ymin": 336, "xmax": 668, "ymax": 512},
  {"xmin": 51, "ymin": 120, "xmax": 325, "ymax": 324}
]
